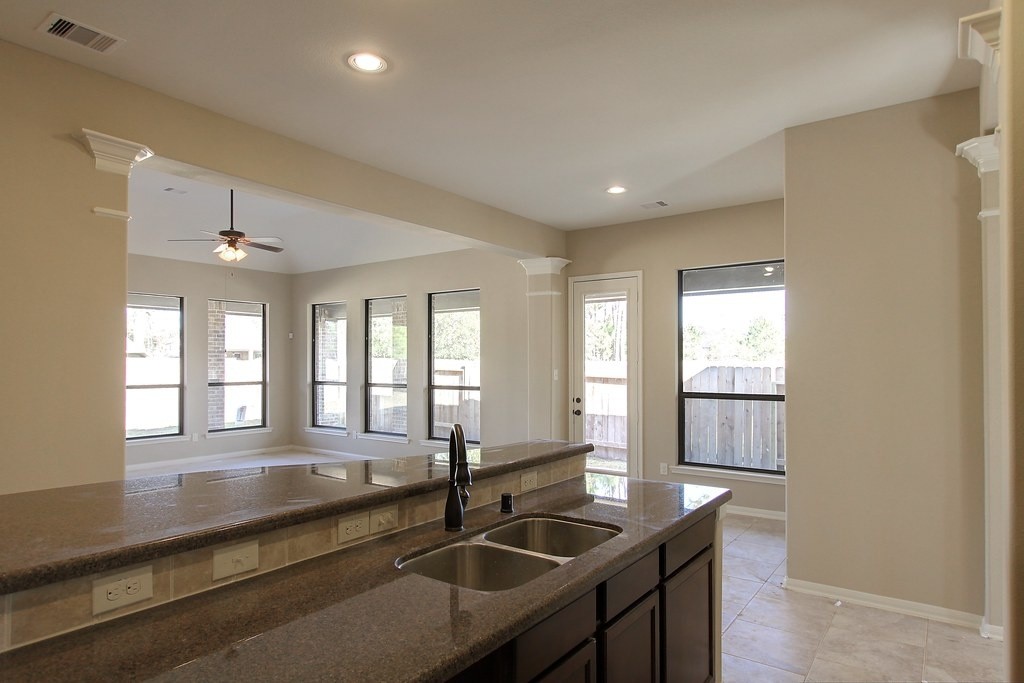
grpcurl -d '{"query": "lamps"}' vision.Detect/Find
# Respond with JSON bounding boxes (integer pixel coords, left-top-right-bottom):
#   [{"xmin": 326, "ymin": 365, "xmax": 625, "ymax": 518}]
[{"xmin": 212, "ymin": 188, "xmax": 250, "ymax": 263}]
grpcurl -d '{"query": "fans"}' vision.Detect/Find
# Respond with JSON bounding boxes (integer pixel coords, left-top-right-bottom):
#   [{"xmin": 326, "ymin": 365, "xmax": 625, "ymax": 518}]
[{"xmin": 165, "ymin": 188, "xmax": 286, "ymax": 255}]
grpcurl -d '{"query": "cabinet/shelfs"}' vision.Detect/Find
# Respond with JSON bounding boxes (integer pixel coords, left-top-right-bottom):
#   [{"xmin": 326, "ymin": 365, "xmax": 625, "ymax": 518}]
[{"xmin": 436, "ymin": 503, "xmax": 721, "ymax": 683}]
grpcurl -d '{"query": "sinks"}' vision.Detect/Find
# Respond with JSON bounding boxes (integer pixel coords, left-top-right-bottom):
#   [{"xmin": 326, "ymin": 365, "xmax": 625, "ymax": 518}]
[
  {"xmin": 394, "ymin": 538, "xmax": 569, "ymax": 593},
  {"xmin": 482, "ymin": 512, "xmax": 625, "ymax": 562}
]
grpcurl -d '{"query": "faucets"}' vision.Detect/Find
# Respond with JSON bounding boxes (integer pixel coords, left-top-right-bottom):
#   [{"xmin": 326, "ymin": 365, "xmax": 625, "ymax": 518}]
[{"xmin": 443, "ymin": 422, "xmax": 474, "ymax": 535}]
